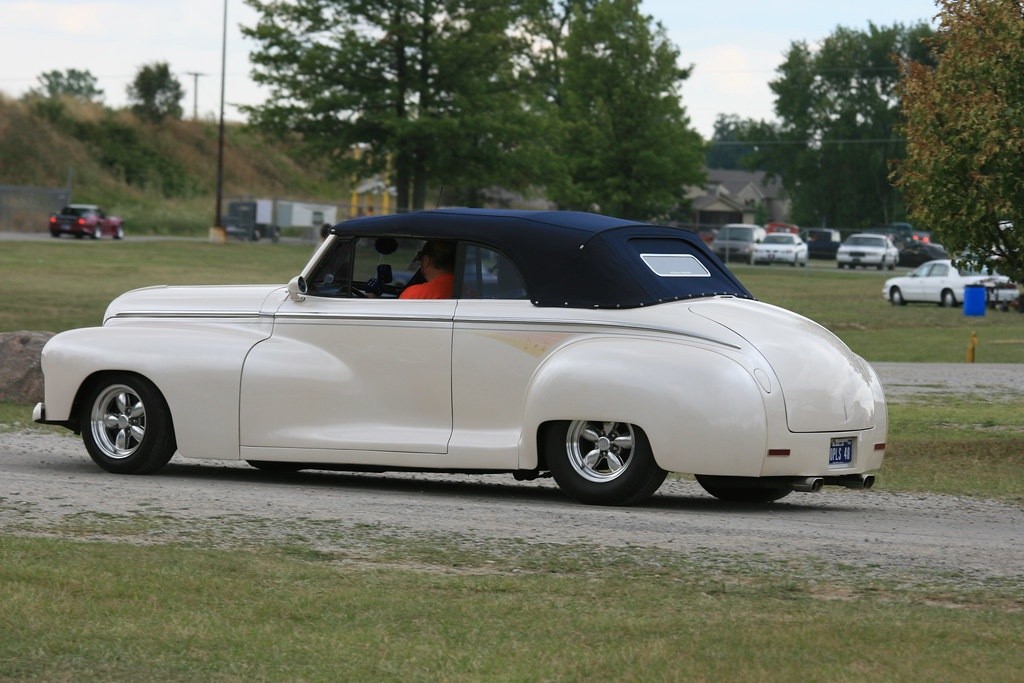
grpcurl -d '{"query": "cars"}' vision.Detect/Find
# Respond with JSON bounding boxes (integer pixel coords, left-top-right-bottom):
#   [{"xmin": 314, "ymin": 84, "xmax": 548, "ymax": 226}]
[
  {"xmin": 48, "ymin": 203, "xmax": 126, "ymax": 240},
  {"xmin": 895, "ymin": 241, "xmax": 950, "ymax": 268},
  {"xmin": 881, "ymin": 257, "xmax": 1021, "ymax": 310},
  {"xmin": 751, "ymin": 231, "xmax": 807, "ymax": 267},
  {"xmin": 836, "ymin": 232, "xmax": 900, "ymax": 273}
]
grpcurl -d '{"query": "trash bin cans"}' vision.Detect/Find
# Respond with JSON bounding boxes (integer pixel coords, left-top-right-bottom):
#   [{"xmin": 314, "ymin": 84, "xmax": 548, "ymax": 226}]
[{"xmin": 963, "ymin": 284, "xmax": 987, "ymax": 317}]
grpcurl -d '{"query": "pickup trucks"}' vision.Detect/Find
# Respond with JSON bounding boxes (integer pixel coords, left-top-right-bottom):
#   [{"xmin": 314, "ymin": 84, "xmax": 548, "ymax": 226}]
[{"xmin": 798, "ymin": 228, "xmax": 842, "ymax": 260}]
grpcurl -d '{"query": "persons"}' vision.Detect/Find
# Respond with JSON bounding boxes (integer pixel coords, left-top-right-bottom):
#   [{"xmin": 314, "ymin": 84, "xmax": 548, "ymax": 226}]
[{"xmin": 367, "ymin": 240, "xmax": 476, "ymax": 299}]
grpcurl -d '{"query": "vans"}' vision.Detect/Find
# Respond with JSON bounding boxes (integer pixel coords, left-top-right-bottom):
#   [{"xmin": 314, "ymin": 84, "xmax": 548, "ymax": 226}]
[{"xmin": 712, "ymin": 222, "xmax": 767, "ymax": 263}]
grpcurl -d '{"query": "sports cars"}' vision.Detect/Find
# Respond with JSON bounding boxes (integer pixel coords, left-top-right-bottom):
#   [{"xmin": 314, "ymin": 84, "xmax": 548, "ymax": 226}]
[{"xmin": 31, "ymin": 207, "xmax": 888, "ymax": 509}]
